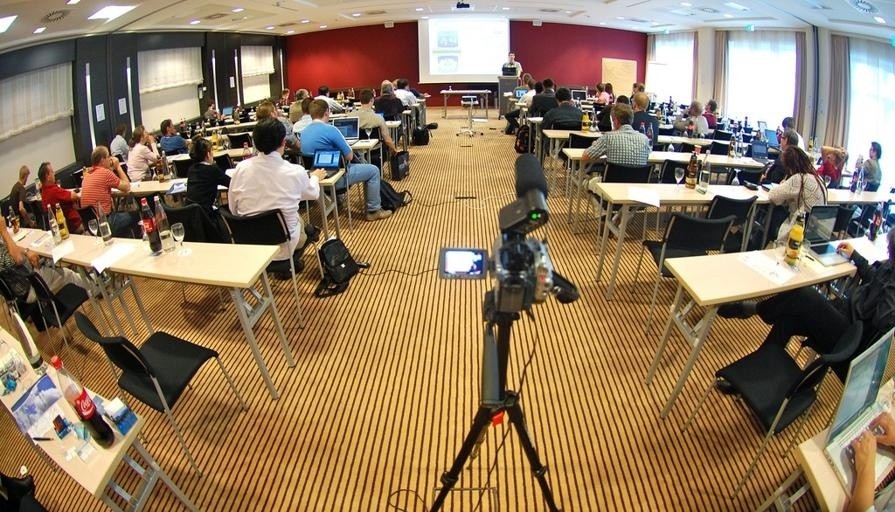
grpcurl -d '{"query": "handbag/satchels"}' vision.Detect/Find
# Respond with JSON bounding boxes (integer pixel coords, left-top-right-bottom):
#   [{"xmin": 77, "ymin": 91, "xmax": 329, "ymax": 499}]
[
  {"xmin": 313, "ymin": 238, "xmax": 359, "ymax": 298},
  {"xmin": 391, "ymin": 151, "xmax": 409, "ymax": 181},
  {"xmin": 412, "ymin": 123, "xmax": 438, "ymax": 145}
]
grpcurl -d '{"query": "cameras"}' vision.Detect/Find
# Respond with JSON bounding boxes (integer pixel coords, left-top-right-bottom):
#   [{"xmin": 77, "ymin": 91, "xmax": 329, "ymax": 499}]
[{"xmin": 438, "ymin": 235, "xmax": 555, "ymax": 313}]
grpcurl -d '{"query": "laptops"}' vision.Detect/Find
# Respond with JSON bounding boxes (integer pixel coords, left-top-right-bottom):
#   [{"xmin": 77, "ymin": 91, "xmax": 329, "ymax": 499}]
[
  {"xmin": 334, "ymin": 117, "xmax": 360, "ymax": 148},
  {"xmin": 222, "ymin": 105, "xmax": 235, "ymax": 120},
  {"xmin": 803, "ymin": 205, "xmax": 850, "ymax": 267},
  {"xmin": 756, "ymin": 120, "xmax": 768, "ymax": 132},
  {"xmin": 308, "ymin": 149, "xmax": 342, "ymax": 179},
  {"xmin": 764, "ymin": 129, "xmax": 781, "ymax": 152},
  {"xmin": 751, "ymin": 141, "xmax": 772, "ymax": 165},
  {"xmin": 822, "ymin": 328, "xmax": 895, "ymax": 512},
  {"xmin": 513, "ymin": 88, "xmax": 529, "ymax": 100},
  {"xmin": 376, "ymin": 110, "xmax": 384, "ymax": 120}
]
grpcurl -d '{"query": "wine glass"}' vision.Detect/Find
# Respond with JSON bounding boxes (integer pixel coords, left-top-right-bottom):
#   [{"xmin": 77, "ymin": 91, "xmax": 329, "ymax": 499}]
[
  {"xmin": 730, "ymin": 168, "xmax": 742, "ymax": 186},
  {"xmin": 150, "ymin": 166, "xmax": 158, "ymax": 183},
  {"xmin": 673, "ymin": 167, "xmax": 685, "ymax": 192},
  {"xmin": 771, "ymin": 240, "xmax": 812, "ymax": 276},
  {"xmin": 364, "ymin": 127, "xmax": 372, "ymax": 142},
  {"xmin": 169, "ymin": 221, "xmax": 192, "ymax": 257},
  {"xmin": 88, "ymin": 219, "xmax": 102, "ymax": 250}
]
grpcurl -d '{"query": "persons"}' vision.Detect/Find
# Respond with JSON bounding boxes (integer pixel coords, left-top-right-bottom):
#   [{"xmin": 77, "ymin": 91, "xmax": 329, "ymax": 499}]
[
  {"xmin": 0, "ymin": 216, "xmax": 110, "ymax": 301},
  {"xmin": 582, "ymin": 103, "xmax": 649, "ymax": 235},
  {"xmin": 299, "ymin": 100, "xmax": 393, "ymax": 220},
  {"xmin": 505, "ymin": 73, "xmax": 582, "ymax": 168},
  {"xmin": 674, "ymin": 100, "xmax": 717, "ymax": 137},
  {"xmin": 350, "ymin": 78, "xmax": 429, "ymax": 162},
  {"xmin": 110, "ymin": 118, "xmax": 187, "ymax": 182},
  {"xmin": 204, "ymin": 100, "xmax": 219, "ymax": 120},
  {"xmin": 595, "ymin": 82, "xmax": 659, "ymax": 147},
  {"xmin": 226, "ymin": 117, "xmax": 327, "ymax": 261},
  {"xmin": 862, "ymin": 141, "xmax": 883, "ymax": 188},
  {"xmin": 718, "ymin": 226, "xmax": 895, "ymax": 393},
  {"xmin": 503, "ymin": 52, "xmax": 522, "ymax": 77},
  {"xmin": 187, "ymin": 137, "xmax": 232, "ymax": 238},
  {"xmin": 257, "ymin": 86, "xmax": 343, "ymax": 150},
  {"xmin": 737, "ymin": 117, "xmax": 849, "ymax": 250},
  {"xmin": 10, "ymin": 146, "xmax": 143, "ymax": 237}
]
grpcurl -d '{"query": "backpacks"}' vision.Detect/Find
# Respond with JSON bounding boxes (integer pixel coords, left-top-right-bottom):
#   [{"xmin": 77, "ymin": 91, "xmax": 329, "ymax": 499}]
[
  {"xmin": 514, "ymin": 124, "xmax": 536, "ymax": 155},
  {"xmin": 379, "ymin": 180, "xmax": 413, "ymax": 212}
]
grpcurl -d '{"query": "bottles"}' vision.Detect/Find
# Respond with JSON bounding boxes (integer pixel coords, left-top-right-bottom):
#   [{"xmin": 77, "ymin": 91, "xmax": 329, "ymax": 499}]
[
  {"xmin": 242, "ymin": 141, "xmax": 257, "ymax": 161},
  {"xmin": 46, "ymin": 203, "xmax": 62, "ymax": 246},
  {"xmin": 49, "ymin": 354, "xmax": 114, "ymax": 449},
  {"xmin": 646, "ymin": 121, "xmax": 654, "ymax": 154},
  {"xmin": 580, "ymin": 107, "xmax": 597, "ymax": 134},
  {"xmin": 786, "ymin": 210, "xmax": 806, "ymax": 267},
  {"xmin": 156, "ymin": 150, "xmax": 172, "ymax": 183},
  {"xmin": 34, "ymin": 178, "xmax": 40, "ymax": 199},
  {"xmin": 685, "ymin": 150, "xmax": 711, "ymax": 195},
  {"xmin": 372, "ymin": 89, "xmax": 377, "ymax": 98},
  {"xmin": 94, "ymin": 199, "xmax": 115, "ymax": 248},
  {"xmin": 151, "ymin": 195, "xmax": 175, "ymax": 254},
  {"xmin": 688, "ymin": 119, "xmax": 694, "ymax": 139},
  {"xmin": 211, "ymin": 129, "xmax": 219, "ymax": 153},
  {"xmin": 6, "ymin": 301, "xmax": 50, "ymax": 376},
  {"xmin": 8, "ymin": 205, "xmax": 20, "ymax": 235},
  {"xmin": 0, "ymin": 339, "xmax": 31, "ymax": 392},
  {"xmin": 55, "ymin": 202, "xmax": 69, "ymax": 241},
  {"xmin": 638, "ymin": 121, "xmax": 645, "ymax": 136},
  {"xmin": 808, "ymin": 137, "xmax": 814, "ymax": 151},
  {"xmin": 179, "ymin": 119, "xmax": 207, "ymax": 138},
  {"xmin": 866, "ymin": 202, "xmax": 884, "ymax": 242},
  {"xmin": 850, "ymin": 168, "xmax": 860, "ymax": 192},
  {"xmin": 335, "ymin": 88, "xmax": 356, "ymax": 106},
  {"xmin": 727, "ymin": 131, "xmax": 744, "ymax": 161},
  {"xmin": 140, "ymin": 197, "xmax": 163, "ymax": 255}
]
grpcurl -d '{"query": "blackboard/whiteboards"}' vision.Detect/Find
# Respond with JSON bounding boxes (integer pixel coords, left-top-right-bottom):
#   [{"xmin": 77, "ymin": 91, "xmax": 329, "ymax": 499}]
[{"xmin": 601, "ymin": 57, "xmax": 637, "ymax": 101}]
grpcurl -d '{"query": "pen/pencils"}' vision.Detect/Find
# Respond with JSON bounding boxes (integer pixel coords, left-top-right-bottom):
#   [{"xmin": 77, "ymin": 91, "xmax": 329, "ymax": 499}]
[
  {"xmin": 33, "ymin": 437, "xmax": 53, "ymax": 440},
  {"xmin": 805, "ymin": 255, "xmax": 814, "ymax": 261}
]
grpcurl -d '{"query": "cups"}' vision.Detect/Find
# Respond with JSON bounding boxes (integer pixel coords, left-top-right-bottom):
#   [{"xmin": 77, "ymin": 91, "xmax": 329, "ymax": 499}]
[
  {"xmin": 824, "ymin": 175, "xmax": 832, "ymax": 187},
  {"xmin": 694, "ymin": 144, "xmax": 702, "ymax": 155},
  {"xmin": 233, "ymin": 112, "xmax": 240, "ymax": 125},
  {"xmin": 7, "ymin": 217, "xmax": 13, "ymax": 234},
  {"xmin": 137, "ymin": 221, "xmax": 149, "ymax": 244}
]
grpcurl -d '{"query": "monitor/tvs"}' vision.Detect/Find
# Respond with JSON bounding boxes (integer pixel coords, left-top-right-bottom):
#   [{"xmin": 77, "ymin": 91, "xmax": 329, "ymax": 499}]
[{"xmin": 572, "ymin": 90, "xmax": 586, "ymax": 101}]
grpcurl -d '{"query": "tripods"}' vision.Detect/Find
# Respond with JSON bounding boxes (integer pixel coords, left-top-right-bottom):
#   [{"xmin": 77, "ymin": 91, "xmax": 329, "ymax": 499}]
[{"xmin": 423, "ymin": 317, "xmax": 565, "ymax": 512}]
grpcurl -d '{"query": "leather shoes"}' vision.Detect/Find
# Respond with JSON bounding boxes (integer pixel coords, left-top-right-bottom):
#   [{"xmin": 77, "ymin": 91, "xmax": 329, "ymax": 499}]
[
  {"xmin": 717, "ymin": 302, "xmax": 751, "ymax": 320},
  {"xmin": 284, "ymin": 258, "xmax": 305, "ymax": 277},
  {"xmin": 365, "ymin": 207, "xmax": 392, "ymax": 221}
]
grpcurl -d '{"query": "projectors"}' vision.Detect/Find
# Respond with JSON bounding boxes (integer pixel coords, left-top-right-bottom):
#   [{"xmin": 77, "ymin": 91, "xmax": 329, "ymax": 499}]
[{"xmin": 456, "ymin": 3, "xmax": 470, "ymax": 8}]
